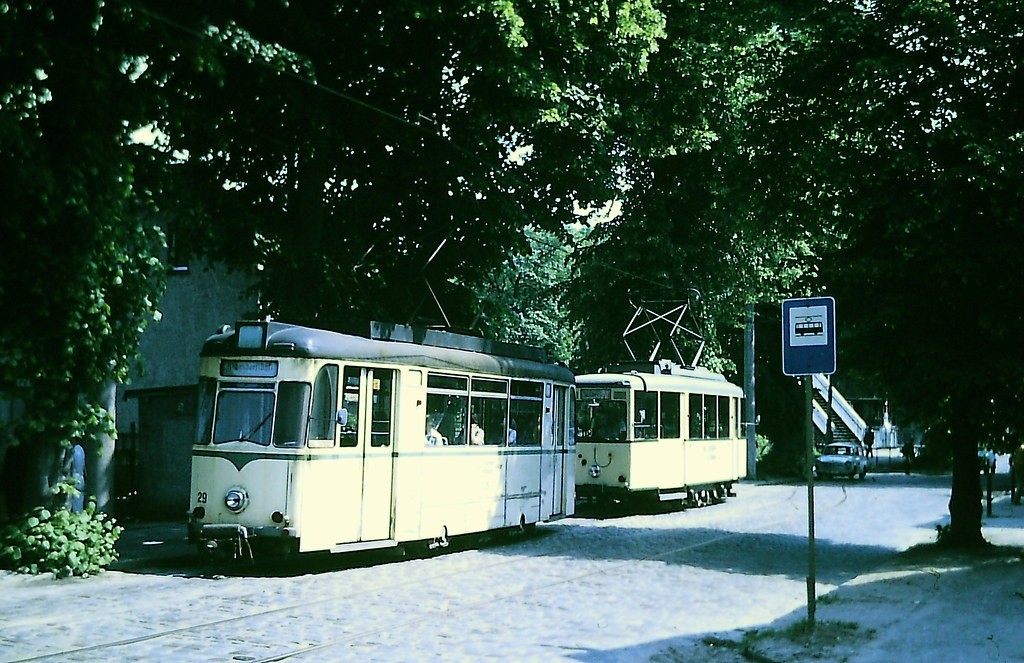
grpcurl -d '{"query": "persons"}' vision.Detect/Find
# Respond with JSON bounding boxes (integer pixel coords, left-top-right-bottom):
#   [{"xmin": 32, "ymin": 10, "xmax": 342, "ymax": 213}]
[
  {"xmin": 864, "ymin": 426, "xmax": 874, "ymax": 461},
  {"xmin": 900, "ymin": 438, "xmax": 915, "ymax": 475},
  {"xmin": 452, "ymin": 412, "xmax": 485, "ymax": 445},
  {"xmin": 499, "ymin": 427, "xmax": 517, "ymax": 447},
  {"xmin": 425, "ymin": 422, "xmax": 443, "ymax": 446},
  {"xmin": 590, "ymin": 402, "xmax": 729, "ymax": 442}
]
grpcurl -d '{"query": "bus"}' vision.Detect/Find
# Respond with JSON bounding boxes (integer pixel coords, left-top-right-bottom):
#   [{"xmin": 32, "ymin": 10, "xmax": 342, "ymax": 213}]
[
  {"xmin": 185, "ymin": 313, "xmax": 581, "ymax": 573},
  {"xmin": 566, "ymin": 354, "xmax": 747, "ymax": 509}
]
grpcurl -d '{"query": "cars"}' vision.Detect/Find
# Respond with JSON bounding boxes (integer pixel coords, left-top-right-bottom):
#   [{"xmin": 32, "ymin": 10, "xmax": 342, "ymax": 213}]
[{"xmin": 817, "ymin": 442, "xmax": 867, "ymax": 480}]
